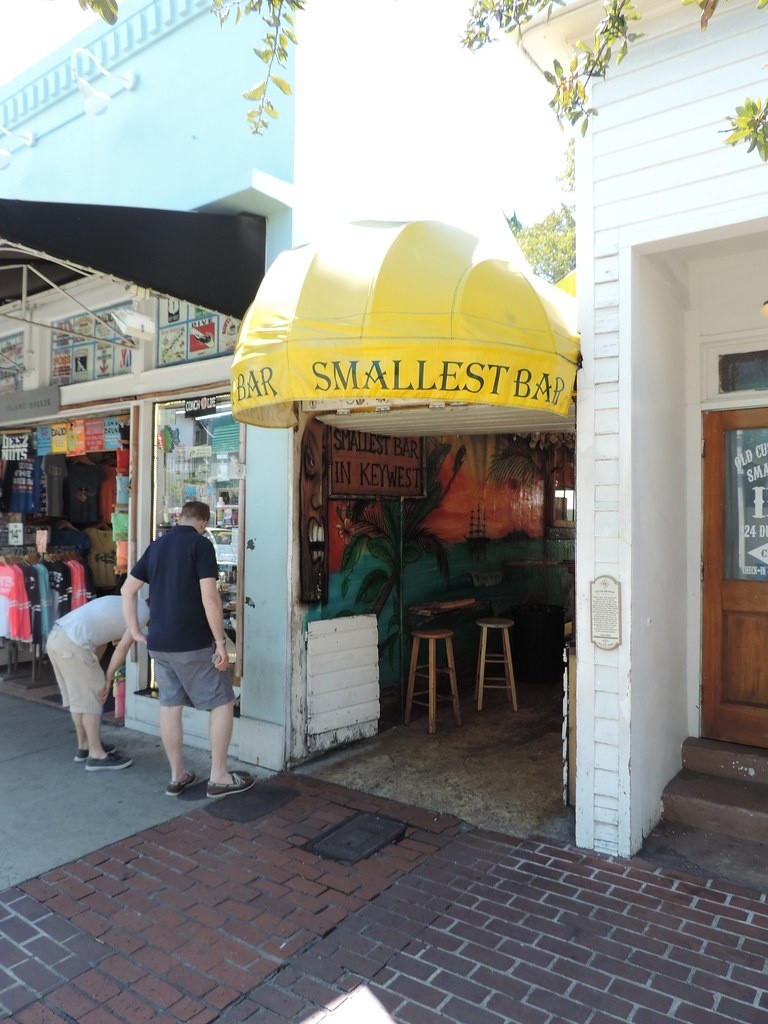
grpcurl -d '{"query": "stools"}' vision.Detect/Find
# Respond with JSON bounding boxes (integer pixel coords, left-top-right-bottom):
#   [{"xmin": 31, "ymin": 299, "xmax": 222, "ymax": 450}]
[
  {"xmin": 473, "ymin": 617, "xmax": 518, "ymax": 711},
  {"xmin": 403, "ymin": 629, "xmax": 461, "ymax": 734}
]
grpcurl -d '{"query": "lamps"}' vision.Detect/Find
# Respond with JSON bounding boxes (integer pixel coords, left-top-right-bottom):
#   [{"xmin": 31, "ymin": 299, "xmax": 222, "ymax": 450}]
[
  {"xmin": 71, "ymin": 48, "xmax": 137, "ymax": 118},
  {"xmin": 0, "ymin": 126, "xmax": 35, "ymax": 169},
  {"xmin": 111, "ymin": 283, "xmax": 155, "ymax": 341}
]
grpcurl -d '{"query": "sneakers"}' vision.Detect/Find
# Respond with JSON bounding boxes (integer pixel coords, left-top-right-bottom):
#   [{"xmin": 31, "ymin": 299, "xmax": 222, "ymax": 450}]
[
  {"xmin": 85, "ymin": 750, "xmax": 133, "ymax": 771},
  {"xmin": 74, "ymin": 740, "xmax": 116, "ymax": 762}
]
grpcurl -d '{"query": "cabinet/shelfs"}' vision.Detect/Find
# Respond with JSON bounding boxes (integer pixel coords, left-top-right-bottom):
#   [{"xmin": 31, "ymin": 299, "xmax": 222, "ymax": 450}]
[{"xmin": 149, "ymin": 392, "xmax": 245, "ymax": 699}]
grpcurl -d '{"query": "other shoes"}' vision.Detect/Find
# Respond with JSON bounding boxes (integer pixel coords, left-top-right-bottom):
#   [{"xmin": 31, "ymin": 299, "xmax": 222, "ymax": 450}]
[
  {"xmin": 165, "ymin": 771, "xmax": 197, "ymax": 796},
  {"xmin": 206, "ymin": 771, "xmax": 255, "ymax": 798}
]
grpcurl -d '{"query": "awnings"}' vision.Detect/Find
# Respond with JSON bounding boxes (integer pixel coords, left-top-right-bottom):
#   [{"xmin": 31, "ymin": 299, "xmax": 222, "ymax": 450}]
[
  {"xmin": 229, "ymin": 217, "xmax": 581, "ymax": 430},
  {"xmin": 1, "ymin": 196, "xmax": 266, "ymax": 350}
]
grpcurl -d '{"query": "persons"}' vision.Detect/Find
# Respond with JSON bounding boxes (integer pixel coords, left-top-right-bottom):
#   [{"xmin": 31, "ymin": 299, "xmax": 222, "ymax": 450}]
[
  {"xmin": 45, "ymin": 595, "xmax": 154, "ymax": 772},
  {"xmin": 120, "ymin": 501, "xmax": 256, "ymax": 799}
]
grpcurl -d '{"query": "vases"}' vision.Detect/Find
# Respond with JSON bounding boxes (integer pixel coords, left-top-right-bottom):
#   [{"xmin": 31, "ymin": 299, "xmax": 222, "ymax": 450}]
[{"xmin": 510, "ymin": 604, "xmax": 568, "ymax": 685}]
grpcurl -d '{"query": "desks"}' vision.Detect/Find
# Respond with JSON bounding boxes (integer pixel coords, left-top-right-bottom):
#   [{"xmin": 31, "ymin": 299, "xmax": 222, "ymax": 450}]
[{"xmin": 408, "ymin": 573, "xmax": 528, "ymax": 627}]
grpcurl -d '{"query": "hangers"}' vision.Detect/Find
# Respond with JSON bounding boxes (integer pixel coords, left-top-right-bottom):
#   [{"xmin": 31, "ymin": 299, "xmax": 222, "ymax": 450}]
[
  {"xmin": 96, "ymin": 521, "xmax": 111, "ymax": 528},
  {"xmin": 0, "ymin": 547, "xmax": 81, "ymax": 567},
  {"xmin": 59, "ymin": 521, "xmax": 77, "ymax": 531},
  {"xmin": 72, "ymin": 455, "xmax": 95, "ymax": 465}
]
